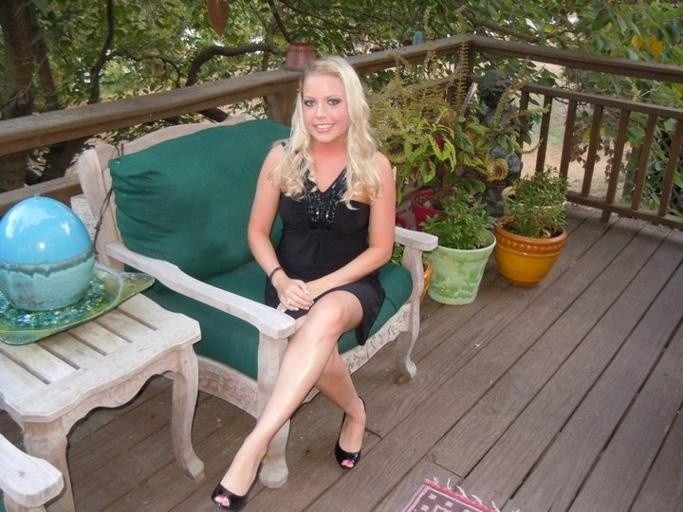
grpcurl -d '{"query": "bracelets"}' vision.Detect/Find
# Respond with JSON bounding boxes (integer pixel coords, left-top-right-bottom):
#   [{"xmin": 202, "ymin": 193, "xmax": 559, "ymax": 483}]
[{"xmin": 267, "ymin": 266, "xmax": 284, "ymax": 288}]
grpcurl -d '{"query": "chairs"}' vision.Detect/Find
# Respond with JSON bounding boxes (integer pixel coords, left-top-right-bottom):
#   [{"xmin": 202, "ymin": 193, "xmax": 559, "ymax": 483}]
[{"xmin": 75, "ymin": 112, "xmax": 439, "ymax": 489}]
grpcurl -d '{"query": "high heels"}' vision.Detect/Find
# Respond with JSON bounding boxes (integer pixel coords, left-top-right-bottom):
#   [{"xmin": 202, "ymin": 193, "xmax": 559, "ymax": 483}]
[
  {"xmin": 211, "ymin": 461, "xmax": 262, "ymax": 511},
  {"xmin": 333, "ymin": 395, "xmax": 366, "ymax": 469}
]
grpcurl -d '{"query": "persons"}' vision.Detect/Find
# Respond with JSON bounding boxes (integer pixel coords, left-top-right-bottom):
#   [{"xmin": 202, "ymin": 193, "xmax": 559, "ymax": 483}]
[{"xmin": 209, "ymin": 56, "xmax": 397, "ymax": 512}]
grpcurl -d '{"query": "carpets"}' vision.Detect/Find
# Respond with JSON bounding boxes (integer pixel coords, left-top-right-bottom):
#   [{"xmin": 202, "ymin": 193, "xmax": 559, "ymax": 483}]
[{"xmin": 400, "ymin": 475, "xmax": 511, "ymax": 512}]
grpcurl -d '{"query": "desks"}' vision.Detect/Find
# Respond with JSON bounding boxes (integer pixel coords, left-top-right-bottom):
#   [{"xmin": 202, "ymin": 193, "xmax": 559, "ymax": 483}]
[{"xmin": 0, "ymin": 290, "xmax": 206, "ymax": 511}]
